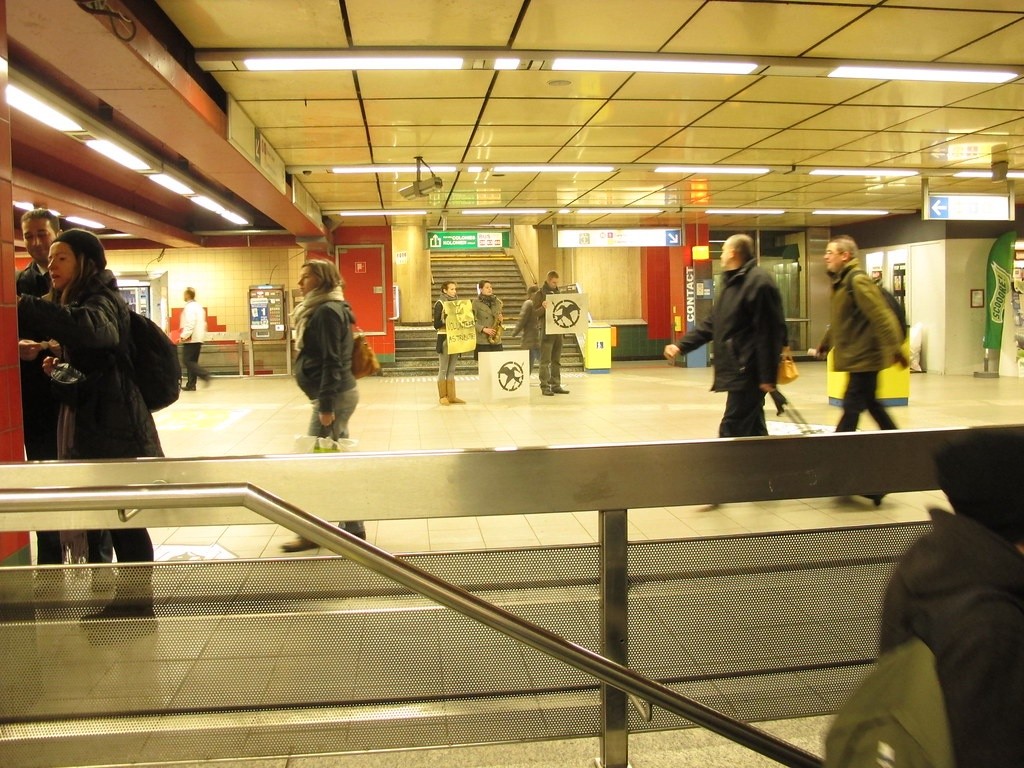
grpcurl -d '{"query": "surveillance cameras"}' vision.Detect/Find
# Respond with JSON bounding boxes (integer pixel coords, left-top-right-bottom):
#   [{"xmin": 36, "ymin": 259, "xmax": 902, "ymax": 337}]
[{"xmin": 398, "ymin": 177, "xmax": 443, "ymax": 200}]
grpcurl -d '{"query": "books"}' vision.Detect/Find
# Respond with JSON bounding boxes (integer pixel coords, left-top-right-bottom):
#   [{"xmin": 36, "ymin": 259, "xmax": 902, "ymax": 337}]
[{"xmin": 170, "ymin": 328, "xmax": 192, "ymax": 344}]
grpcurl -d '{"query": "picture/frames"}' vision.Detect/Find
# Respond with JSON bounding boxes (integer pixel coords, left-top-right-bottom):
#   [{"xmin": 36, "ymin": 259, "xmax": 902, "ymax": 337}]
[{"xmin": 970, "ymin": 289, "xmax": 985, "ymax": 308}]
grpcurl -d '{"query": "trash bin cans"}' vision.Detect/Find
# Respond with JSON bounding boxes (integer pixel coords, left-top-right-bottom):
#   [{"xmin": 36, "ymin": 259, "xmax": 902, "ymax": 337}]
[{"xmin": 584, "ymin": 322, "xmax": 612, "ymax": 374}]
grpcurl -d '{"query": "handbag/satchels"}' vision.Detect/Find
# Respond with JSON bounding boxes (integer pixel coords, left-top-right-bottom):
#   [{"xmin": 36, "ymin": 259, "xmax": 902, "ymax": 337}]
[
  {"xmin": 777, "ymin": 346, "xmax": 801, "ymax": 385},
  {"xmin": 350, "ymin": 322, "xmax": 380, "ymax": 379},
  {"xmin": 289, "ymin": 419, "xmax": 364, "ymax": 454}
]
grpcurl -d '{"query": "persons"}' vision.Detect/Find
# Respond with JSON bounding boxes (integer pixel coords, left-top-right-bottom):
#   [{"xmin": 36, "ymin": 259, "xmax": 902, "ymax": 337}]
[
  {"xmin": 180, "ymin": 287, "xmax": 212, "ymax": 391},
  {"xmin": 433, "ymin": 281, "xmax": 465, "ymax": 406},
  {"xmin": 473, "ymin": 280, "xmax": 503, "ymax": 361},
  {"xmin": 0, "ymin": 208, "xmax": 164, "ymax": 645},
  {"xmin": 280, "ymin": 259, "xmax": 365, "ymax": 551},
  {"xmin": 882, "ymin": 431, "xmax": 1024, "ymax": 768},
  {"xmin": 663, "ymin": 233, "xmax": 788, "ymax": 508},
  {"xmin": 512, "ymin": 285, "xmax": 542, "ymax": 375},
  {"xmin": 533, "ymin": 271, "xmax": 570, "ymax": 396},
  {"xmin": 814, "ymin": 234, "xmax": 909, "ymax": 433},
  {"xmin": 770, "ymin": 328, "xmax": 791, "ymax": 416}
]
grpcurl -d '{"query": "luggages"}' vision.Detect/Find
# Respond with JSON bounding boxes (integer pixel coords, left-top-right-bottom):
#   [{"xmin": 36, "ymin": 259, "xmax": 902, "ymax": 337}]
[{"xmin": 769, "ymin": 386, "xmax": 888, "ymax": 506}]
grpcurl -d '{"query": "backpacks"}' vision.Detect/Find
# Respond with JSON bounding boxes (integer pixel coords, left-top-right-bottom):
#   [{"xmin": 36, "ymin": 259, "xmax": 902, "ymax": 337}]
[
  {"xmin": 821, "ymin": 585, "xmax": 1024, "ymax": 768},
  {"xmin": 119, "ymin": 308, "xmax": 183, "ymax": 412},
  {"xmin": 843, "ymin": 270, "xmax": 908, "ymax": 346}
]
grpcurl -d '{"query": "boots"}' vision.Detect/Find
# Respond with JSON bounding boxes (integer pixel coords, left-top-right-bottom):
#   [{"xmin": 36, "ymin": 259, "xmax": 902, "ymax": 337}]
[
  {"xmin": 447, "ymin": 380, "xmax": 467, "ymax": 403},
  {"xmin": 437, "ymin": 380, "xmax": 450, "ymax": 406}
]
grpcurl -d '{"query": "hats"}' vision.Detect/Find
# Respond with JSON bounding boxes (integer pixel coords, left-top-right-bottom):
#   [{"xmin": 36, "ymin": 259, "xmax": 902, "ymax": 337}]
[
  {"xmin": 48, "ymin": 229, "xmax": 110, "ymax": 273},
  {"xmin": 928, "ymin": 429, "xmax": 1024, "ymax": 530}
]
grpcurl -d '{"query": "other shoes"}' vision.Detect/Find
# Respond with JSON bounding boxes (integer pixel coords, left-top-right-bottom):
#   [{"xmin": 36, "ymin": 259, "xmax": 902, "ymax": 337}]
[
  {"xmin": 542, "ymin": 389, "xmax": 554, "ymax": 396},
  {"xmin": 181, "ymin": 386, "xmax": 196, "ymax": 392},
  {"xmin": 552, "ymin": 389, "xmax": 570, "ymax": 394},
  {"xmin": 92, "ymin": 572, "xmax": 116, "ymax": 591},
  {"xmin": 352, "ymin": 530, "xmax": 366, "ymax": 540},
  {"xmin": 32, "ymin": 571, "xmax": 68, "ymax": 602},
  {"xmin": 203, "ymin": 376, "xmax": 212, "ymax": 383},
  {"xmin": 281, "ymin": 535, "xmax": 319, "ymax": 551},
  {"xmin": 77, "ymin": 590, "xmax": 158, "ymax": 629}
]
